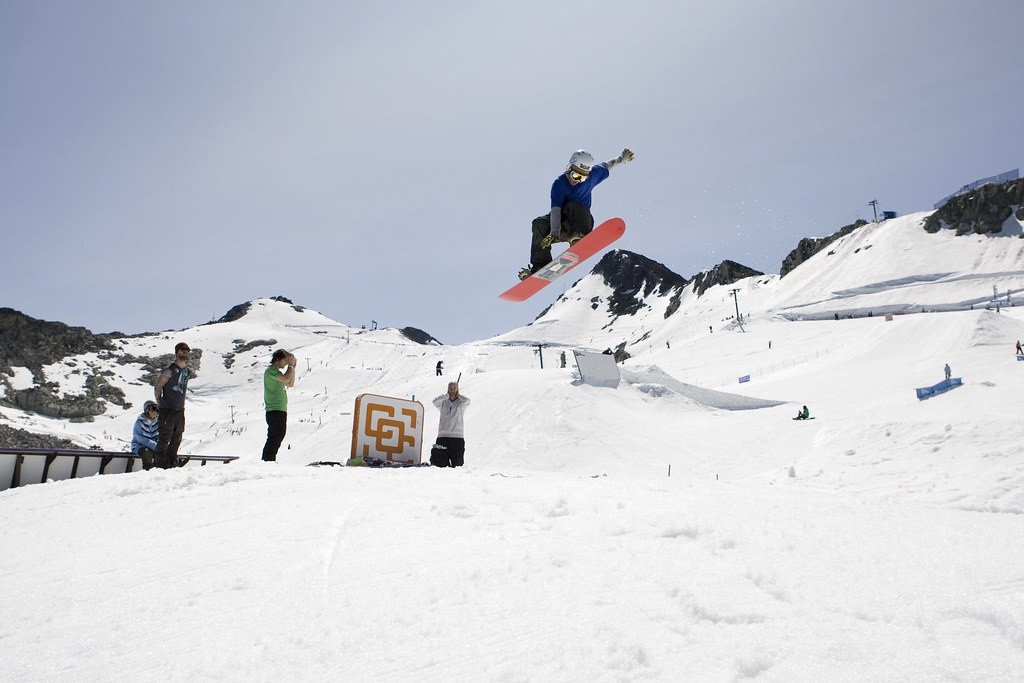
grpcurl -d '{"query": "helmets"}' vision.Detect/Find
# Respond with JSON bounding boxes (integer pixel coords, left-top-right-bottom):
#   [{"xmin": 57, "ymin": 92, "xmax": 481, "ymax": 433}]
[{"xmin": 567, "ymin": 149, "xmax": 594, "ymax": 176}]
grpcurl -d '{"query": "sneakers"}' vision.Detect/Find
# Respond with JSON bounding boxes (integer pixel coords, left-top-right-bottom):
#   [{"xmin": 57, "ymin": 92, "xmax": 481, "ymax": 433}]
[{"xmin": 517, "ymin": 266, "xmax": 536, "ymax": 281}]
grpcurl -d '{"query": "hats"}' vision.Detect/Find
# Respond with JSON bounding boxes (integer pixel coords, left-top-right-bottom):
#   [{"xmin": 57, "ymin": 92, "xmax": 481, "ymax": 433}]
[
  {"xmin": 270, "ymin": 348, "xmax": 293, "ymax": 364},
  {"xmin": 175, "ymin": 342, "xmax": 189, "ymax": 355},
  {"xmin": 143, "ymin": 400, "xmax": 157, "ymax": 412}
]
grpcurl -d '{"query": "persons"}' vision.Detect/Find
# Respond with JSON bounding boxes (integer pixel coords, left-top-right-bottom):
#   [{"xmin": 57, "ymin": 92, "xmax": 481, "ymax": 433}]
[
  {"xmin": 153, "ymin": 341, "xmax": 190, "ymax": 467},
  {"xmin": 944, "ymin": 364, "xmax": 951, "ymax": 381},
  {"xmin": 260, "ymin": 349, "xmax": 297, "ymax": 461},
  {"xmin": 1015, "ymin": 340, "xmax": 1024, "ymax": 355},
  {"xmin": 797, "ymin": 405, "xmax": 809, "ymax": 420},
  {"xmin": 133, "ymin": 399, "xmax": 189, "ymax": 469},
  {"xmin": 529, "ymin": 147, "xmax": 635, "ymax": 270},
  {"xmin": 435, "ymin": 360, "xmax": 442, "ymax": 376},
  {"xmin": 288, "ymin": 365, "xmax": 292, "ymax": 368},
  {"xmin": 433, "ymin": 382, "xmax": 470, "ymax": 468}
]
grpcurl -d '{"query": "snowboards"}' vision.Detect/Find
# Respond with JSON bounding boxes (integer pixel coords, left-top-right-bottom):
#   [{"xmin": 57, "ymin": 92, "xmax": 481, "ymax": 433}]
[{"xmin": 498, "ymin": 217, "xmax": 626, "ymax": 302}]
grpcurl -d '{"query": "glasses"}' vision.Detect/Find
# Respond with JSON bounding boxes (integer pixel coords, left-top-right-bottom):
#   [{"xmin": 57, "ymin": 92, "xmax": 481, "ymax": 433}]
[
  {"xmin": 569, "ymin": 169, "xmax": 588, "ymax": 183},
  {"xmin": 180, "ymin": 347, "xmax": 190, "ymax": 352}
]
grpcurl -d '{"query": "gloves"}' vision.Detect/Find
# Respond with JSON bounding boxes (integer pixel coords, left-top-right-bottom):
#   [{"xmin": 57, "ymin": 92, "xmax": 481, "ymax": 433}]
[
  {"xmin": 541, "ymin": 233, "xmax": 556, "ymax": 250},
  {"xmin": 622, "ymin": 147, "xmax": 634, "ymax": 162}
]
